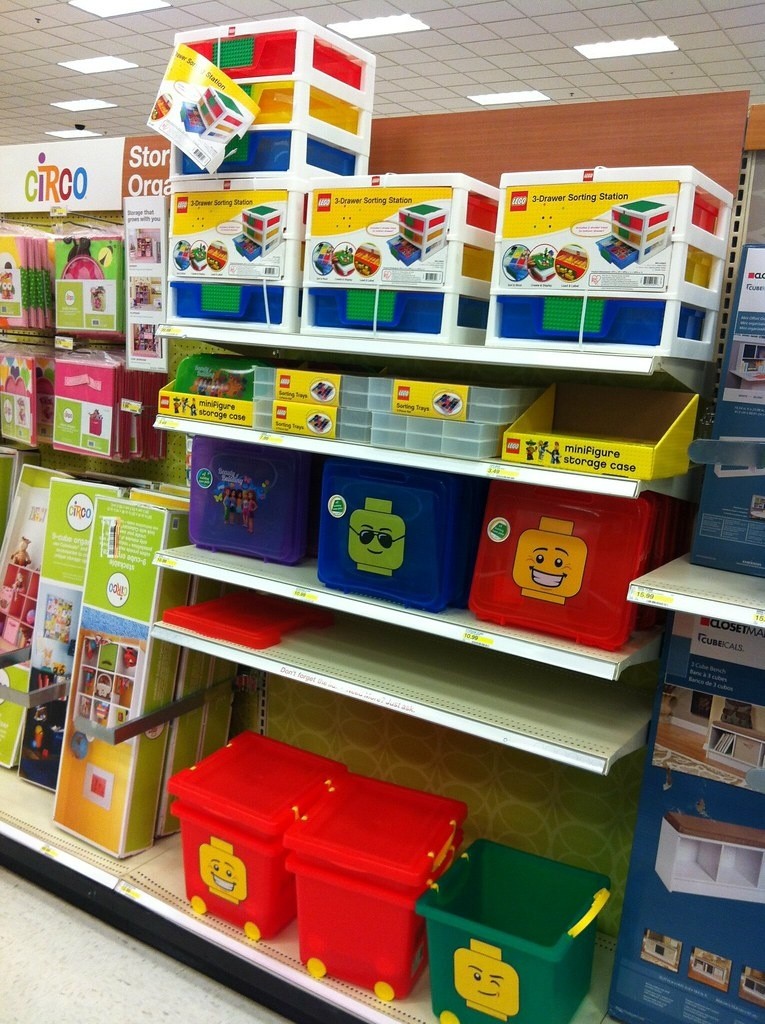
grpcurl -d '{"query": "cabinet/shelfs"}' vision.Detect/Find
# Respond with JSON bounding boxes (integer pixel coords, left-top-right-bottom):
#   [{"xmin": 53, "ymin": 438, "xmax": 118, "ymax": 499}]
[
  {"xmin": 0, "ymin": 130, "xmax": 265, "ymax": 926},
  {"xmin": 111, "ymin": 87, "xmax": 749, "ymax": 1024},
  {"xmin": 603, "ymin": 97, "xmax": 764, "ymax": 1023}
]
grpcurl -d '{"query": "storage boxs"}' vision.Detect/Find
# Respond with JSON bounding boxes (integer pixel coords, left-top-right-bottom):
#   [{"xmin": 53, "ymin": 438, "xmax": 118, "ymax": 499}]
[
  {"xmin": 164, "ymin": 729, "xmax": 349, "ymax": 938},
  {"xmin": 284, "ymin": 771, "xmax": 469, "ymax": 1000},
  {"xmin": 416, "ymin": 839, "xmax": 610, "ymax": 1024},
  {"xmin": 0, "ymin": 443, "xmax": 239, "ymax": 860},
  {"xmin": 158, "ymin": 16, "xmax": 734, "ymax": 650}
]
